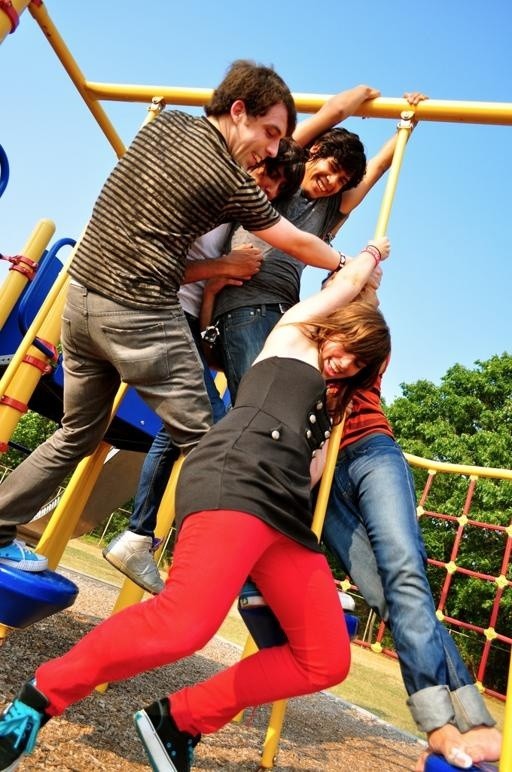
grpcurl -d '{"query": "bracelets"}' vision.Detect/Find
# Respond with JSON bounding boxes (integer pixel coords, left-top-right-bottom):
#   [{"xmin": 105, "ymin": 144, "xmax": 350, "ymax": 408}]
[
  {"xmin": 360, "ymin": 244, "xmax": 382, "ymax": 267},
  {"xmin": 335, "ymin": 250, "xmax": 345, "ymax": 271}
]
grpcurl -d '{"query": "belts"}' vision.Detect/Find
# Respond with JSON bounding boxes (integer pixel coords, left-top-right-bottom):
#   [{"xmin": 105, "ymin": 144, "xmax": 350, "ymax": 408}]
[{"xmin": 264, "ymin": 303, "xmax": 288, "ymax": 315}]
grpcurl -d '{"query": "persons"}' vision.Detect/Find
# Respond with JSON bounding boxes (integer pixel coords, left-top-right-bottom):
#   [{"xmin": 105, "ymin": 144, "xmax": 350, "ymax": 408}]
[
  {"xmin": 211, "ymin": 84, "xmax": 430, "ymax": 413},
  {"xmin": 2, "ymin": 232, "xmax": 390, "ymax": 772},
  {"xmin": 105, "ymin": 130, "xmax": 310, "ymax": 611},
  {"xmin": 0, "ymin": 57, "xmax": 385, "ymax": 585},
  {"xmin": 320, "ymin": 265, "xmax": 504, "ymax": 770}
]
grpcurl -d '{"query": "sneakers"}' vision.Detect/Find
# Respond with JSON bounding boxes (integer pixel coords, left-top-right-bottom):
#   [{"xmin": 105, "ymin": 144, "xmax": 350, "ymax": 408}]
[
  {"xmin": 337, "ymin": 591, "xmax": 355, "ymax": 610},
  {"xmin": 237, "ymin": 577, "xmax": 269, "ymax": 608},
  {"xmin": 0, "ymin": 540, "xmax": 49, "ymax": 571},
  {"xmin": 133, "ymin": 698, "xmax": 202, "ymax": 771},
  {"xmin": 0, "ymin": 676, "xmax": 52, "ymax": 771},
  {"xmin": 103, "ymin": 532, "xmax": 164, "ymax": 594}
]
